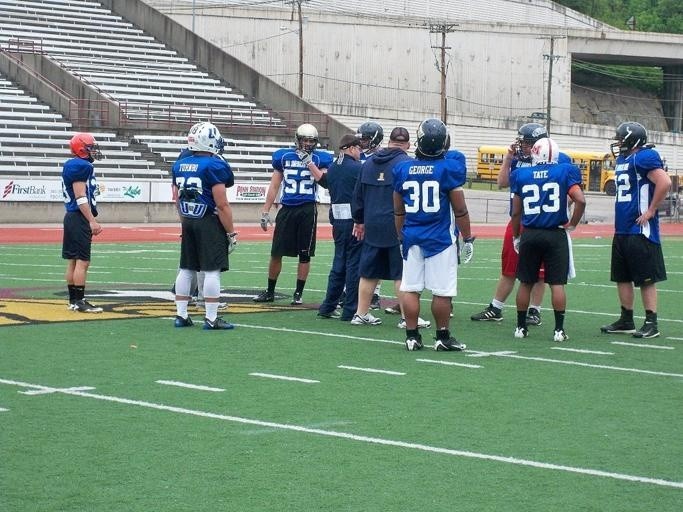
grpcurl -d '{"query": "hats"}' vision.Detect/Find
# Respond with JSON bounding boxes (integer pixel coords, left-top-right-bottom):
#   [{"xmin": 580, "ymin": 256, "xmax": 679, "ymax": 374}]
[
  {"xmin": 337, "ymin": 134, "xmax": 363, "ymax": 149},
  {"xmin": 391, "ymin": 127, "xmax": 409, "ymax": 142}
]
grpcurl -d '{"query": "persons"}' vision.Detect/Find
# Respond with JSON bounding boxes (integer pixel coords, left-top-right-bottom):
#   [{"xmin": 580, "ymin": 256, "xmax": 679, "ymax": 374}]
[
  {"xmin": 62, "ymin": 133, "xmax": 105, "ymax": 312},
  {"xmin": 600, "ymin": 120, "xmax": 671, "ymax": 338},
  {"xmin": 509, "ymin": 138, "xmax": 587, "ymax": 344},
  {"xmin": 472, "ymin": 123, "xmax": 572, "ymax": 327},
  {"xmin": 169, "ymin": 121, "xmax": 238, "ymax": 331}
]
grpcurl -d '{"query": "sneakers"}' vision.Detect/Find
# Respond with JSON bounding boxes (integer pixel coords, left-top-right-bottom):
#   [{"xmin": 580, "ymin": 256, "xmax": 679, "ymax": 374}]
[
  {"xmin": 252, "ymin": 290, "xmax": 274, "ymax": 302},
  {"xmin": 75, "ymin": 299, "xmax": 104, "ymax": 313},
  {"xmin": 316, "ymin": 294, "xmax": 453, "ymax": 328},
  {"xmin": 171, "ymin": 284, "xmax": 234, "ymax": 330},
  {"xmin": 470, "ymin": 303, "xmax": 569, "ymax": 342},
  {"xmin": 405, "ymin": 335, "xmax": 423, "ymax": 351},
  {"xmin": 290, "ymin": 292, "xmax": 302, "ymax": 305},
  {"xmin": 633, "ymin": 321, "xmax": 659, "ymax": 338},
  {"xmin": 67, "ymin": 303, "xmax": 76, "ymax": 310},
  {"xmin": 600, "ymin": 320, "xmax": 635, "ymax": 334}
]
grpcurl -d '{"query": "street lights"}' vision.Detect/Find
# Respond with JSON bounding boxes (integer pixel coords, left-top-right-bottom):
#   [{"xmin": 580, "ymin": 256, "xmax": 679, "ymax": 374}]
[
  {"xmin": 545, "ymin": 73, "xmax": 563, "ymax": 137},
  {"xmin": 279, "ymin": 25, "xmax": 304, "ymax": 97}
]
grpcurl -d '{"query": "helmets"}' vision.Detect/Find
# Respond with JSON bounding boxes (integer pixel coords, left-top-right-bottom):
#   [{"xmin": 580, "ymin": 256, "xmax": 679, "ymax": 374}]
[
  {"xmin": 414, "ymin": 118, "xmax": 449, "ymax": 159},
  {"xmin": 529, "ymin": 138, "xmax": 559, "ymax": 166},
  {"xmin": 70, "ymin": 133, "xmax": 103, "ymax": 162},
  {"xmin": 187, "ymin": 121, "xmax": 225, "ymax": 156},
  {"xmin": 512, "ymin": 123, "xmax": 548, "ymax": 162},
  {"xmin": 294, "ymin": 124, "xmax": 318, "ymax": 153},
  {"xmin": 610, "ymin": 121, "xmax": 647, "ymax": 158},
  {"xmin": 356, "ymin": 122, "xmax": 383, "ymax": 155}
]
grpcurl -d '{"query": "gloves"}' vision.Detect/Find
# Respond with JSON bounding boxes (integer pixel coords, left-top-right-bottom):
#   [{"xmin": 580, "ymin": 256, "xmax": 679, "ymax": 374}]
[
  {"xmin": 513, "ymin": 236, "xmax": 521, "ymax": 253},
  {"xmin": 295, "ymin": 148, "xmax": 313, "ymax": 165},
  {"xmin": 227, "ymin": 231, "xmax": 237, "ymax": 253},
  {"xmin": 461, "ymin": 237, "xmax": 473, "ymax": 265},
  {"xmin": 260, "ymin": 212, "xmax": 273, "ymax": 231}
]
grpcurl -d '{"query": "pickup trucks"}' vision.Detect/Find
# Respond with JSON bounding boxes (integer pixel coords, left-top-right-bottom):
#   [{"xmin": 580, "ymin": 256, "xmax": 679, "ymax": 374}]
[{"xmin": 518, "ymin": 112, "xmax": 551, "ymax": 119}]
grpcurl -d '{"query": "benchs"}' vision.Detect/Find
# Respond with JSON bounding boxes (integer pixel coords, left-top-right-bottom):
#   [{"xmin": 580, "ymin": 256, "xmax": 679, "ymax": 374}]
[
  {"xmin": 0, "ymin": 109, "xmax": 334, "ymax": 179},
  {"xmin": 0, "ymin": 62, "xmax": 268, "ymax": 110},
  {"xmin": 2, "ymin": 1, "xmax": 191, "ymax": 60}
]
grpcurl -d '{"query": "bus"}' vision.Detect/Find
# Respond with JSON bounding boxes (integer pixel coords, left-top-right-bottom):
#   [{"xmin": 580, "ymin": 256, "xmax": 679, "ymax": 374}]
[{"xmin": 473, "ymin": 144, "xmax": 680, "ymax": 197}]
